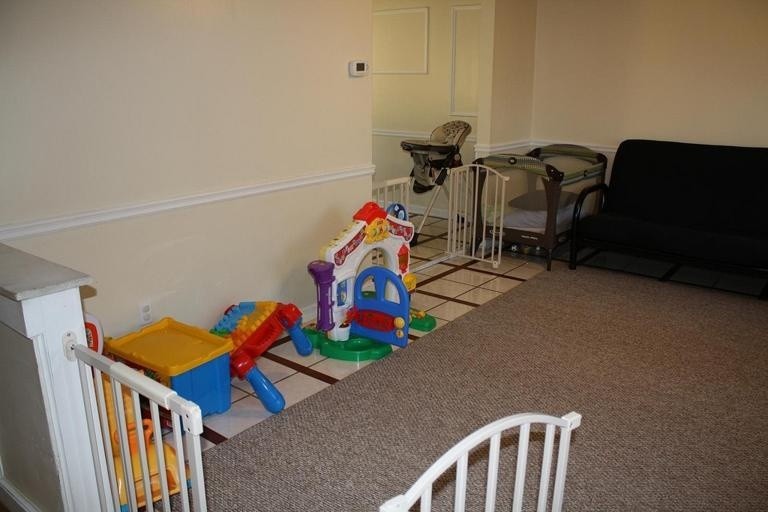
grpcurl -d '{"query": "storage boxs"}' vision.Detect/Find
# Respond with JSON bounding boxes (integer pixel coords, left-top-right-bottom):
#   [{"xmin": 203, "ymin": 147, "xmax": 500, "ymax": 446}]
[{"xmin": 104, "ymin": 317, "xmax": 235, "ymax": 435}]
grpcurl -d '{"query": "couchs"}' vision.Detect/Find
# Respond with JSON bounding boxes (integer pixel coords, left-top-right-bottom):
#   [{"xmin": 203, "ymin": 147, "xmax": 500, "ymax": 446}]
[{"xmin": 567, "ymin": 139, "xmax": 767, "ymax": 301}]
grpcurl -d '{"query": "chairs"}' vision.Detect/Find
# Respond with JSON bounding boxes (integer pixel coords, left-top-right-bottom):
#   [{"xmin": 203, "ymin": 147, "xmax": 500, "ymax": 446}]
[{"xmin": 401, "ymin": 121, "xmax": 471, "ymax": 247}]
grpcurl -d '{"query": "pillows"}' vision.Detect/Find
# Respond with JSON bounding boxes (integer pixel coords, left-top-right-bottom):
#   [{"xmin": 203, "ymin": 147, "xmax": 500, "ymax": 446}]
[{"xmin": 499, "ymin": 188, "xmax": 587, "ymax": 229}]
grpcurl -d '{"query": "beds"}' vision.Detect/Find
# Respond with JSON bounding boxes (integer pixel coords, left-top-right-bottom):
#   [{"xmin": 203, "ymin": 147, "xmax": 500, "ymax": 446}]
[{"xmin": 470, "ymin": 144, "xmax": 607, "ymax": 272}]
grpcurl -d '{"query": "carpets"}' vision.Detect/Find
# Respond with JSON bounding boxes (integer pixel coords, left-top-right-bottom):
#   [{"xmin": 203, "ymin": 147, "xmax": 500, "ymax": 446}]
[{"xmin": 136, "ymin": 261, "xmax": 767, "ymax": 512}]
